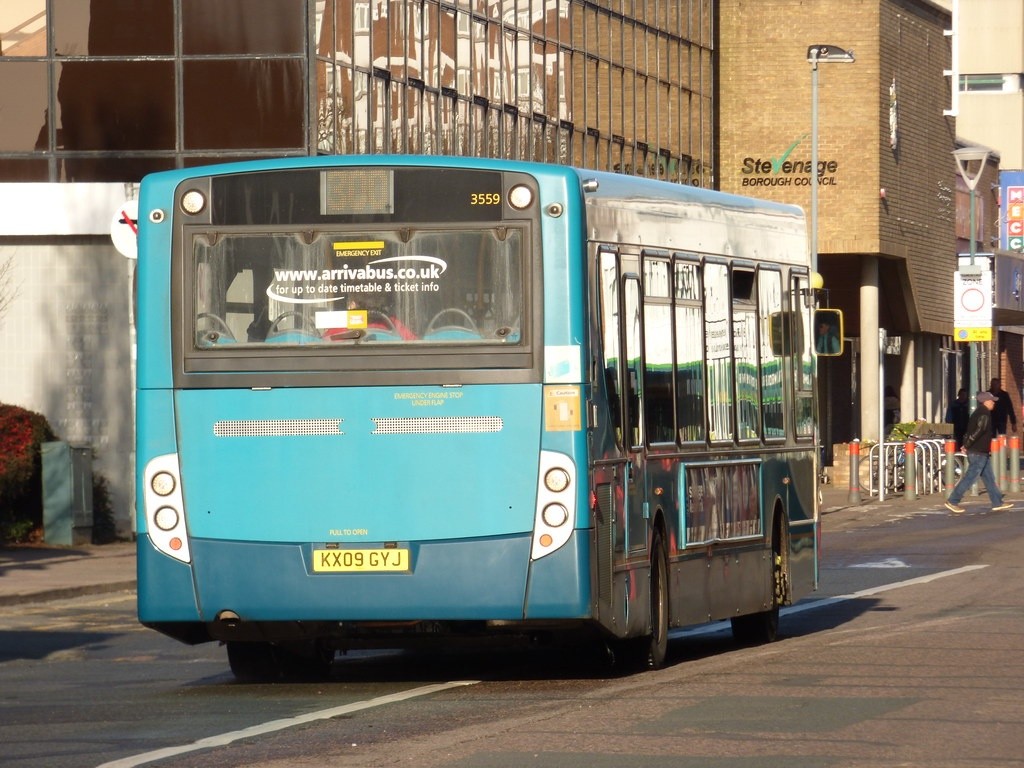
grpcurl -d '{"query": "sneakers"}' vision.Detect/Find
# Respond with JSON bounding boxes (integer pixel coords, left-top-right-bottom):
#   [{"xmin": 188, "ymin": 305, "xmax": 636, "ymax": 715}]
[
  {"xmin": 945, "ymin": 501, "xmax": 965, "ymax": 513},
  {"xmin": 992, "ymin": 502, "xmax": 1014, "ymax": 512}
]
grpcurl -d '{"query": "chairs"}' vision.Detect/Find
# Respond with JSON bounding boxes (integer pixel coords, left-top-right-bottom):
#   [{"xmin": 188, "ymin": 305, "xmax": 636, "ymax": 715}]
[{"xmin": 196, "ymin": 306, "xmax": 521, "ymax": 344}]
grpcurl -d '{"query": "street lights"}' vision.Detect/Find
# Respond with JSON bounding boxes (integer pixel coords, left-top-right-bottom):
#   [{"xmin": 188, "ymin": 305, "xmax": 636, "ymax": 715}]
[
  {"xmin": 951, "ymin": 147, "xmax": 992, "ymax": 497},
  {"xmin": 807, "ymin": 45, "xmax": 855, "ymax": 271}
]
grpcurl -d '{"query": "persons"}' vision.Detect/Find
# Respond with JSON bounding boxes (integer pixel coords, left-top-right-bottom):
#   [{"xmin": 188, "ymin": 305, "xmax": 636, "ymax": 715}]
[
  {"xmin": 945, "ymin": 392, "xmax": 1014, "ymax": 512},
  {"xmin": 946, "ymin": 388, "xmax": 969, "ymax": 452},
  {"xmin": 985, "ymin": 378, "xmax": 1017, "ymax": 438}
]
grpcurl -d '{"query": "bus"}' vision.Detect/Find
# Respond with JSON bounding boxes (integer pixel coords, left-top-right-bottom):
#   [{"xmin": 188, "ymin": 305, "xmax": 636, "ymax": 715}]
[{"xmin": 136, "ymin": 154, "xmax": 842, "ymax": 680}]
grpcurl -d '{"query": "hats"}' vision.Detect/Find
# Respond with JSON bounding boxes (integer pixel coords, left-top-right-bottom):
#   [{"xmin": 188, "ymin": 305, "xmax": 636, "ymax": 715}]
[{"xmin": 976, "ymin": 392, "xmax": 998, "ymax": 403}]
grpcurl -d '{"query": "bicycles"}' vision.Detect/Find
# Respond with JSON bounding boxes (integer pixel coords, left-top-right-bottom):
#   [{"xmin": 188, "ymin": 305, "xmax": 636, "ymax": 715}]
[{"xmin": 858, "ymin": 427, "xmax": 964, "ymax": 495}]
